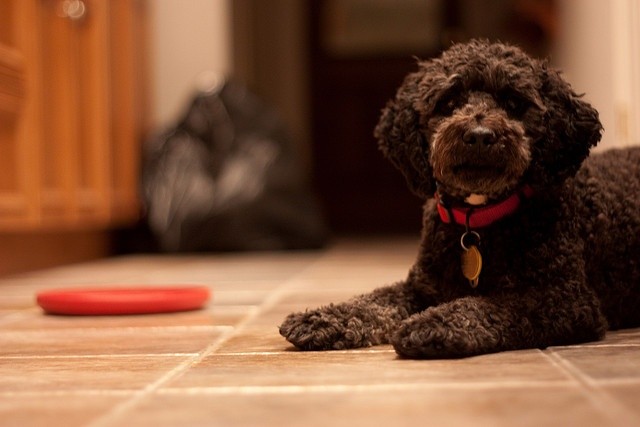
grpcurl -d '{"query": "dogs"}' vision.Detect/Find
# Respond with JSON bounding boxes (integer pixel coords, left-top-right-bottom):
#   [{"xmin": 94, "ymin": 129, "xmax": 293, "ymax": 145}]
[{"xmin": 279, "ymin": 38, "xmax": 636, "ymax": 362}]
